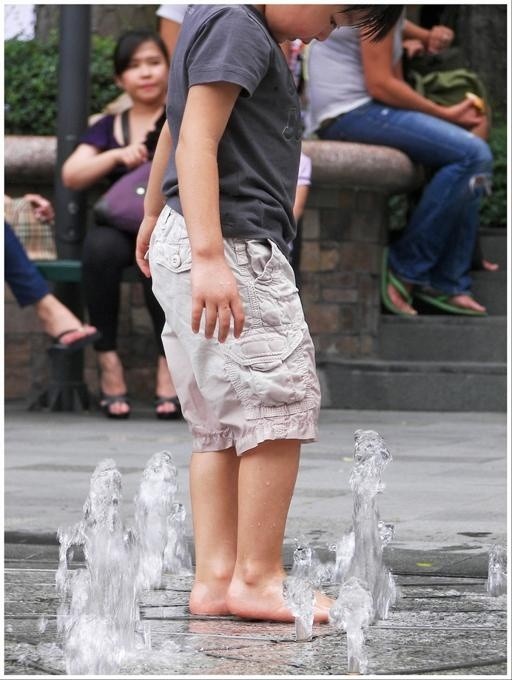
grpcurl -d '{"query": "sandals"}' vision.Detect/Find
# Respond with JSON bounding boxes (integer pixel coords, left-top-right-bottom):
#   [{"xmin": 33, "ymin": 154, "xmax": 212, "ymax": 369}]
[
  {"xmin": 98, "ymin": 387, "xmax": 131, "ymax": 420},
  {"xmin": 153, "ymin": 392, "xmax": 182, "ymax": 420}
]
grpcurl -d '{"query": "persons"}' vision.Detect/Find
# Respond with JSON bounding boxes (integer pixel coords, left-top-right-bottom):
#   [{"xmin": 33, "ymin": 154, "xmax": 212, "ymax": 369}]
[
  {"xmin": 85, "ymin": 4, "xmax": 182, "ymax": 130},
  {"xmin": 270, "ymin": 6, "xmax": 492, "ymax": 315},
  {"xmin": 138, "ymin": 4, "xmax": 410, "ymax": 628},
  {"xmin": 58, "ymin": 25, "xmax": 185, "ymax": 425},
  {"xmin": 3, "ymin": 187, "xmax": 100, "ymax": 362}
]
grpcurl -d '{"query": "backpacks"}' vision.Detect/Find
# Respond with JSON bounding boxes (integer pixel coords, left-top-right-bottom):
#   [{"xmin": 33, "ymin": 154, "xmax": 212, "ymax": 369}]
[{"xmin": 400, "ymin": 64, "xmax": 494, "ymax": 140}]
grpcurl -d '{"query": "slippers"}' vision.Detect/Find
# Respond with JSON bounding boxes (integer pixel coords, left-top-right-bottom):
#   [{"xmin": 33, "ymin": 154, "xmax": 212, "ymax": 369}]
[
  {"xmin": 413, "ymin": 287, "xmax": 491, "ymax": 317},
  {"xmin": 45, "ymin": 321, "xmax": 103, "ymax": 356},
  {"xmin": 379, "ymin": 245, "xmax": 414, "ymax": 318}
]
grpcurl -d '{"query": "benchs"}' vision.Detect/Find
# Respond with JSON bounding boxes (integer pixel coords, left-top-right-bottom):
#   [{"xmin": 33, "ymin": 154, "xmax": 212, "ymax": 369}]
[{"xmin": 32, "ymin": 261, "xmax": 143, "ymax": 286}]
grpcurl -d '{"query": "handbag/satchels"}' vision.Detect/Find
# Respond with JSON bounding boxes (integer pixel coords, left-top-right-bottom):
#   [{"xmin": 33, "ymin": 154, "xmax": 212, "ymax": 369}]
[
  {"xmin": 91, "ymin": 157, "xmax": 154, "ymax": 238},
  {"xmin": 4, "ymin": 195, "xmax": 60, "ymax": 263}
]
